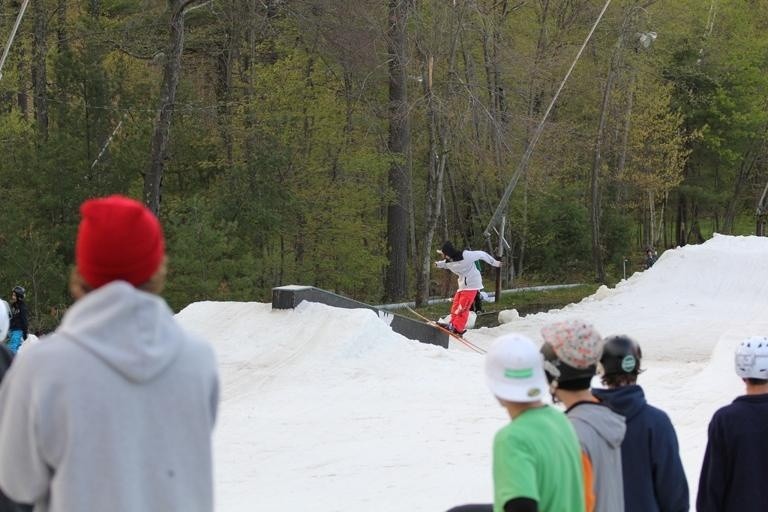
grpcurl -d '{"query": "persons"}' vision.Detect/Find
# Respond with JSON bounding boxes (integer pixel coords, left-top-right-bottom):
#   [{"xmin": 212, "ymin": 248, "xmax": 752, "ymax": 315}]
[
  {"xmin": 696, "ymin": 335, "xmax": 768, "ymax": 512},
  {"xmin": 645, "ymin": 249, "xmax": 659, "ymax": 269},
  {"xmin": 0, "ymin": 195, "xmax": 220, "ymax": 512},
  {"xmin": 482, "ymin": 335, "xmax": 590, "ymax": 512},
  {"xmin": 6, "ymin": 286, "xmax": 28, "ymax": 351},
  {"xmin": 433, "ymin": 241, "xmax": 506, "ymax": 337},
  {"xmin": 538, "ymin": 317, "xmax": 628, "ymax": 512},
  {"xmin": 591, "ymin": 334, "xmax": 689, "ymax": 512}
]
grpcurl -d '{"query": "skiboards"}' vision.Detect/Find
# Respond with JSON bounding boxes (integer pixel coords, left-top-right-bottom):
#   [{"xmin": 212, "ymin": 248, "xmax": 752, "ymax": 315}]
[{"xmin": 405, "ymin": 304, "xmax": 487, "ymax": 355}]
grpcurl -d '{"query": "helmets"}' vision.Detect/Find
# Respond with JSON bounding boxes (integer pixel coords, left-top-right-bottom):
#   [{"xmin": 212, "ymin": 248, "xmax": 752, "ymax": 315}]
[
  {"xmin": 540, "ymin": 317, "xmax": 603, "ymax": 370},
  {"xmin": 12, "ymin": 286, "xmax": 25, "ymax": 298},
  {"xmin": 734, "ymin": 335, "xmax": 767, "ymax": 380},
  {"xmin": 602, "ymin": 334, "xmax": 642, "ymax": 376}
]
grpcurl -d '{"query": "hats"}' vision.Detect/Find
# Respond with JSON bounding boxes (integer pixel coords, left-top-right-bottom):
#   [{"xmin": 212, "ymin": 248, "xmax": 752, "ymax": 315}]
[
  {"xmin": 76, "ymin": 194, "xmax": 163, "ymax": 285},
  {"xmin": 479, "ymin": 332, "xmax": 549, "ymax": 403}
]
